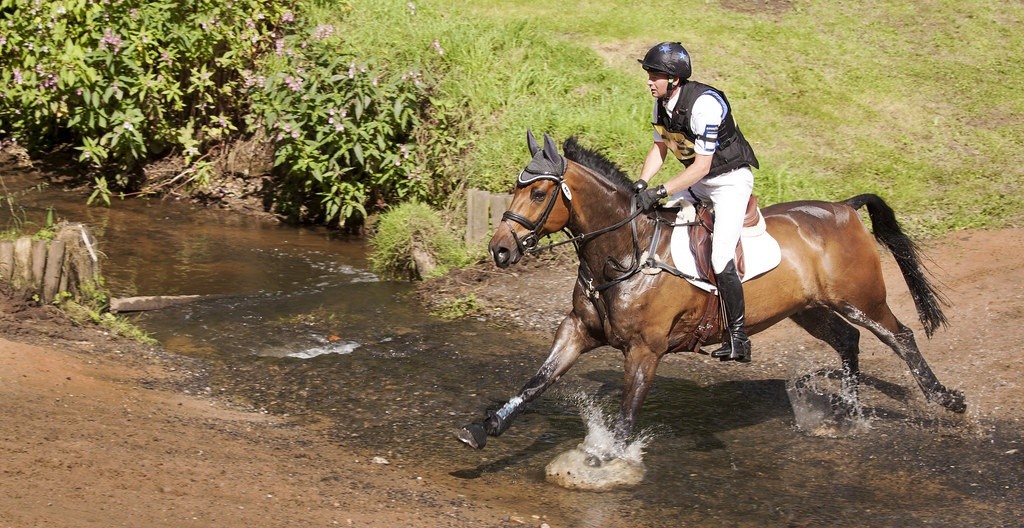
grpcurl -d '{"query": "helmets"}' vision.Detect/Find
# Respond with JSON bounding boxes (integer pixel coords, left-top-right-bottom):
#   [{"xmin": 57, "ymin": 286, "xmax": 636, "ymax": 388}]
[{"xmin": 638, "ymin": 42, "xmax": 691, "ymax": 78}]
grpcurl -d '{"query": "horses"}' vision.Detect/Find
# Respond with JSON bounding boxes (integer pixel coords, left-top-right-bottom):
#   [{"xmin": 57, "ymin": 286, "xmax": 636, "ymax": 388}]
[{"xmin": 453, "ymin": 129, "xmax": 967, "ymax": 469}]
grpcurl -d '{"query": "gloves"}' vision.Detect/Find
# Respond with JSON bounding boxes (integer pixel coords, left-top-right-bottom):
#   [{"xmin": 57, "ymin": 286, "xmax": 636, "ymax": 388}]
[
  {"xmin": 633, "ymin": 179, "xmax": 648, "ymax": 191},
  {"xmin": 638, "ymin": 185, "xmax": 668, "ymax": 210}
]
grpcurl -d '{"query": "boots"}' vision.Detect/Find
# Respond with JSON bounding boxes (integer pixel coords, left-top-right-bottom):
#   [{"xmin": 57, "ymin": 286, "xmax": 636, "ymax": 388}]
[{"xmin": 711, "ymin": 258, "xmax": 751, "ymax": 363}]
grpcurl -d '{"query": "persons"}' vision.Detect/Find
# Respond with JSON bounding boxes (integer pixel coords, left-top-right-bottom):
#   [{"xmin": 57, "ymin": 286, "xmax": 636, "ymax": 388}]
[{"xmin": 633, "ymin": 42, "xmax": 759, "ymax": 362}]
